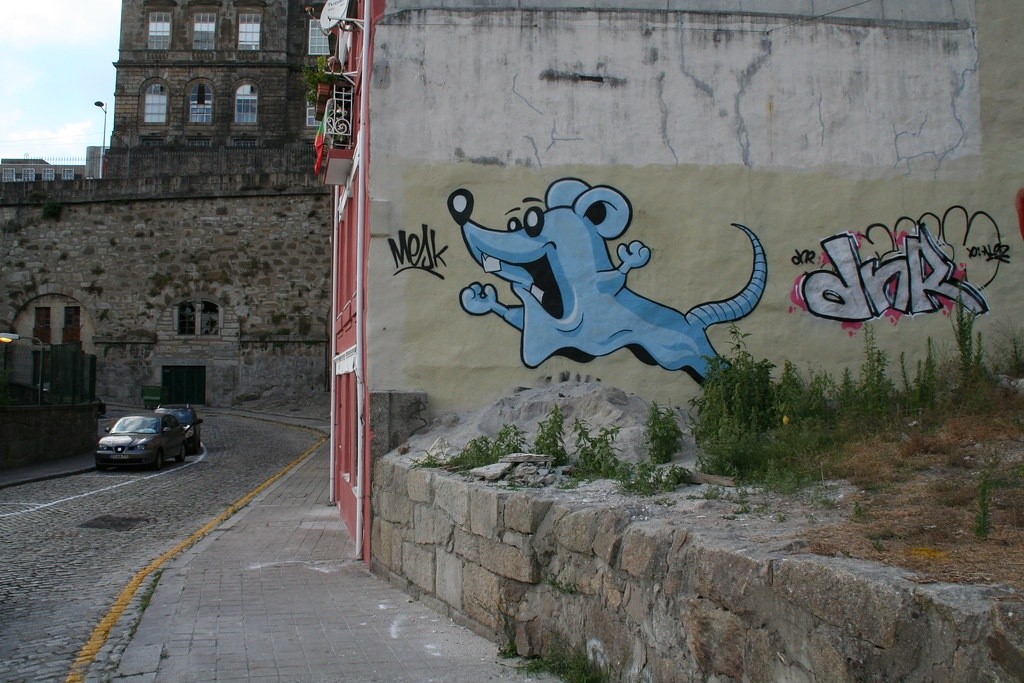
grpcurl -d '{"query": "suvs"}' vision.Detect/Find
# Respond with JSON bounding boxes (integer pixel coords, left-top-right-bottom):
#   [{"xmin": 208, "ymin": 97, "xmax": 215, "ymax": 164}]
[{"xmin": 154, "ymin": 403, "xmax": 204, "ymax": 455}]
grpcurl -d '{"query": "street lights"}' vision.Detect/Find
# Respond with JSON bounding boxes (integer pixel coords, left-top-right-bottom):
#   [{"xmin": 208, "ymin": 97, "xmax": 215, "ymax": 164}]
[
  {"xmin": 94, "ymin": 101, "xmax": 107, "ymax": 177},
  {"xmin": 1, "ymin": 332, "xmax": 44, "ymax": 405}
]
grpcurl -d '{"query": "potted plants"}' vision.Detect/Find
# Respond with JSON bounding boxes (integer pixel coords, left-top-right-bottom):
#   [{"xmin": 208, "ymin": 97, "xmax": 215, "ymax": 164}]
[{"xmin": 304, "ymin": 56, "xmax": 347, "ymax": 121}]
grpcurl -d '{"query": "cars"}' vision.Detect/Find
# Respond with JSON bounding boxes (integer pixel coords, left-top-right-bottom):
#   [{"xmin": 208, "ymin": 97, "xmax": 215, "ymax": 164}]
[{"xmin": 94, "ymin": 412, "xmax": 186, "ymax": 470}]
